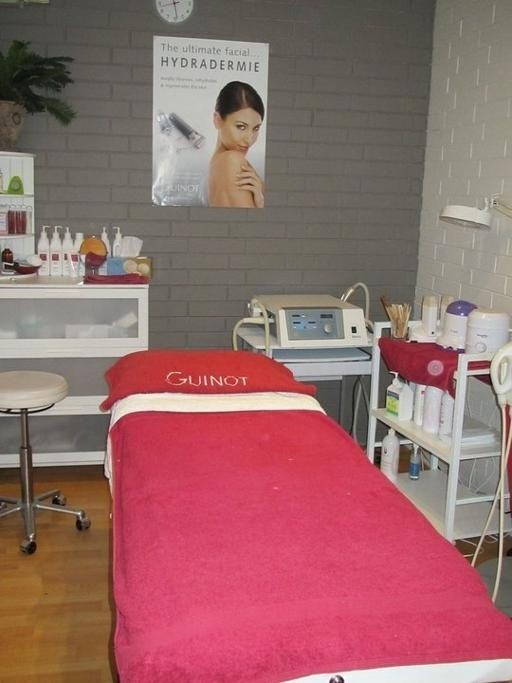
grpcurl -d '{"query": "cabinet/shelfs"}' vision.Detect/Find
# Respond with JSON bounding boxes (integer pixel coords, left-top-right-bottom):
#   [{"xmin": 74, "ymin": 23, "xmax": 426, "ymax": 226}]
[
  {"xmin": 364, "ymin": 316, "xmax": 512, "ymax": 544},
  {"xmin": 1, "ymin": 148, "xmax": 39, "ymax": 266},
  {"xmin": 1, "ymin": 269, "xmax": 150, "ymax": 471}
]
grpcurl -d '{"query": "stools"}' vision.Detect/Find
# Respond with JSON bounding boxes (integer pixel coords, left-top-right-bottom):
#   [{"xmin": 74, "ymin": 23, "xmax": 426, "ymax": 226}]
[{"xmin": 0, "ymin": 369, "xmax": 94, "ymax": 556}]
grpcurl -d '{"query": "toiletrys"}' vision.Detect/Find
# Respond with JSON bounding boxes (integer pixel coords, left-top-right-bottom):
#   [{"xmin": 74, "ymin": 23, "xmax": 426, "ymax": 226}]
[
  {"xmin": 37, "ymin": 224, "xmax": 122, "ymax": 277},
  {"xmin": 2, "ymin": 201, "xmax": 35, "ymax": 235},
  {"xmin": 409, "ymin": 443, "xmax": 420, "ymax": 480}
]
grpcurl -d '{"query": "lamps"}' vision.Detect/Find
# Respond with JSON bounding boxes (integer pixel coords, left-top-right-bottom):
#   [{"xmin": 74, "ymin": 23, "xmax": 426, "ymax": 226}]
[{"xmin": 435, "ymin": 194, "xmax": 511, "ymax": 233}]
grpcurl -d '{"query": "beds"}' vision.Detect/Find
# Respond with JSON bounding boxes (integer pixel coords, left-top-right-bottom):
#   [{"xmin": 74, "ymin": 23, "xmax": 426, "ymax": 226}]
[{"xmin": 101, "ymin": 347, "xmax": 511, "ymax": 683}]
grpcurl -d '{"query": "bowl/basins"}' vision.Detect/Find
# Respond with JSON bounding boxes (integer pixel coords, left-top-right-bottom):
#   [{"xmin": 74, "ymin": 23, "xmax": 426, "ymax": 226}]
[{"xmin": 13, "ymin": 262, "xmax": 42, "ymax": 274}]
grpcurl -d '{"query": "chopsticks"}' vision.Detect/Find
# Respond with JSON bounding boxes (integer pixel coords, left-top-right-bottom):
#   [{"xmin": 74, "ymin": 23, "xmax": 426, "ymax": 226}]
[{"xmin": 384, "ymin": 302, "xmax": 412, "ymax": 339}]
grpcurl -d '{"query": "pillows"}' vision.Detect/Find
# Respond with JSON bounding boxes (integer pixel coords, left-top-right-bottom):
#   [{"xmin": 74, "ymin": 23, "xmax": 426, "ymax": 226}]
[{"xmin": 101, "ymin": 351, "xmax": 315, "ymax": 409}]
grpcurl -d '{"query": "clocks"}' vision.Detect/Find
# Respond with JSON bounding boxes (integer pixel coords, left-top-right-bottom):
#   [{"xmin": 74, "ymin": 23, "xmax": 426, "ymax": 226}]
[{"xmin": 155, "ymin": 0, "xmax": 192, "ymax": 24}]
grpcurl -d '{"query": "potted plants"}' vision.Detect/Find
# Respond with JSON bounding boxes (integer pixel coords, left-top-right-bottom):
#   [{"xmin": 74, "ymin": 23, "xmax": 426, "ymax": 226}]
[{"xmin": 0, "ymin": 38, "xmax": 81, "ymax": 147}]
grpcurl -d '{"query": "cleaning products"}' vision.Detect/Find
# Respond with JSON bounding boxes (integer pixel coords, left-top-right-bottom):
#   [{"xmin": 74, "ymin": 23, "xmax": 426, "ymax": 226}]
[{"xmin": 386, "ymin": 371, "xmax": 455, "ymax": 441}]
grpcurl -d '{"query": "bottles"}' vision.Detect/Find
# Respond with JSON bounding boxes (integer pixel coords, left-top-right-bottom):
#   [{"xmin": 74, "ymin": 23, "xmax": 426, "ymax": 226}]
[
  {"xmin": 1, "ymin": 246, "xmax": 13, "ymax": 272},
  {"xmin": 421, "ymin": 293, "xmax": 456, "ymax": 336},
  {"xmin": 0, "ymin": 203, "xmax": 33, "ymax": 236},
  {"xmin": 398, "ymin": 383, "xmax": 415, "ymax": 422},
  {"xmin": 0, "ymin": 168, "xmax": 4, "ymax": 193},
  {"xmin": 409, "ymin": 379, "xmax": 455, "ymax": 435},
  {"xmin": 73, "ymin": 231, "xmax": 85, "ymax": 251}
]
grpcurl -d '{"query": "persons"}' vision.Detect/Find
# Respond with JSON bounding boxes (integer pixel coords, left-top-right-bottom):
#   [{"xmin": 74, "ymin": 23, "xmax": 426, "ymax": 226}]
[{"xmin": 210, "ymin": 80, "xmax": 267, "ymax": 207}]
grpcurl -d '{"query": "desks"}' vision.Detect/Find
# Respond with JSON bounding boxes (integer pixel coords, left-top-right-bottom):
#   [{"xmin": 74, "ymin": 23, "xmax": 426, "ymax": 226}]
[{"xmin": 234, "ymin": 324, "xmax": 372, "ymax": 384}]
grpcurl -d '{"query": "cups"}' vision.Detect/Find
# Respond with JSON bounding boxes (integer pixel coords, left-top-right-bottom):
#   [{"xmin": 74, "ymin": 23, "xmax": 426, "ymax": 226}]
[{"xmin": 390, "ymin": 318, "xmax": 408, "ymax": 342}]
